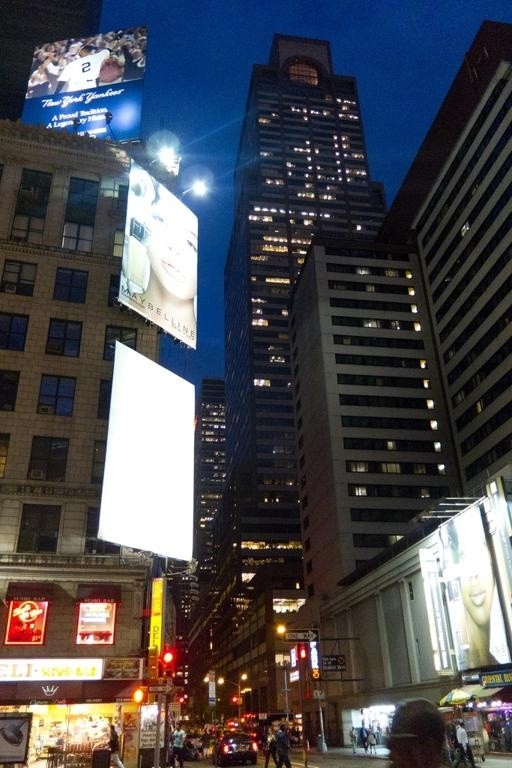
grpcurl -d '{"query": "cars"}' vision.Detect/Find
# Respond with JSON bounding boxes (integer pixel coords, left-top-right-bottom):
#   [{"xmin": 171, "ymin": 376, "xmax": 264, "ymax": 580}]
[{"xmin": 181, "ymin": 726, "xmax": 258, "ymax": 768}]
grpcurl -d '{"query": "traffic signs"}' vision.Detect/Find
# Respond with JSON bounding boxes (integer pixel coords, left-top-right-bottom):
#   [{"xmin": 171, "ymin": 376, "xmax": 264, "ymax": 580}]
[
  {"xmin": 283, "ymin": 629, "xmax": 320, "ymax": 642},
  {"xmin": 149, "ymin": 685, "xmax": 174, "ymax": 693}
]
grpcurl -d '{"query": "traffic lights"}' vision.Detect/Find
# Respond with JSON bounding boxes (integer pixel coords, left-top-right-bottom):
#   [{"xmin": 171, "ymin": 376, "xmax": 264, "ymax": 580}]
[
  {"xmin": 160, "ymin": 645, "xmax": 175, "ymax": 669},
  {"xmin": 136, "ymin": 686, "xmax": 155, "ymax": 704}
]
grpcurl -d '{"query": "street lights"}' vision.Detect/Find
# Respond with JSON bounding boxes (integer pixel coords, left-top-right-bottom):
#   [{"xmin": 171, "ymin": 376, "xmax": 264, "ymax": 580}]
[
  {"xmin": 278, "ymin": 659, "xmax": 293, "ymax": 752},
  {"xmin": 218, "ymin": 673, "xmax": 248, "ymax": 727}
]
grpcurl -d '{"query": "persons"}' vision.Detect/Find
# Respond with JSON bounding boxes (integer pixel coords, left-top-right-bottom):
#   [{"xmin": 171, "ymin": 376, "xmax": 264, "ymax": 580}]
[
  {"xmin": 107, "ymin": 725, "xmax": 125, "ymax": 768},
  {"xmin": 444, "ymin": 717, "xmax": 478, "ymax": 768},
  {"xmin": 201, "ymin": 727, "xmax": 221, "ymax": 767},
  {"xmin": 171, "ymin": 722, "xmax": 186, "ymax": 768},
  {"xmin": 387, "ymin": 696, "xmax": 452, "ymax": 768},
  {"xmin": 264, "ymin": 725, "xmax": 301, "ymax": 767},
  {"xmin": 349, "ymin": 722, "xmax": 377, "ymax": 754},
  {"xmin": 445, "ymin": 509, "xmax": 501, "ymax": 670},
  {"xmin": 129, "ymin": 183, "xmax": 196, "ymax": 343},
  {"xmin": 27, "ymin": 28, "xmax": 147, "ymax": 100}
]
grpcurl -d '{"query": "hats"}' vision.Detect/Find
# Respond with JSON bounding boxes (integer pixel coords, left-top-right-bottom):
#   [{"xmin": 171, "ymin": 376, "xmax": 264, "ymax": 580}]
[{"xmin": 383, "ymin": 697, "xmax": 445, "ymax": 743}]
